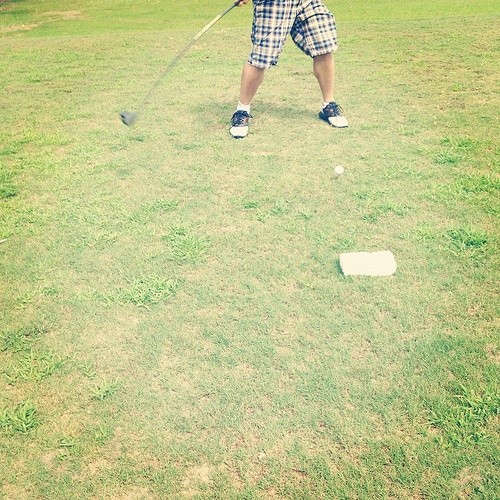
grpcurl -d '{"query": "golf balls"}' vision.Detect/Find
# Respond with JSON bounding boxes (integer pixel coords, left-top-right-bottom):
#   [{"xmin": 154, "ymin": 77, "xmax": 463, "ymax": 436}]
[{"xmin": 335, "ymin": 166, "xmax": 344, "ymax": 174}]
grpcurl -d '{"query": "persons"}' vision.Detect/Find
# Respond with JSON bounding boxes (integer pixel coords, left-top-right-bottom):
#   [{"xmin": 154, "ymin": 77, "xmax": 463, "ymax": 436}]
[{"xmin": 225, "ymin": 1, "xmax": 348, "ymax": 140}]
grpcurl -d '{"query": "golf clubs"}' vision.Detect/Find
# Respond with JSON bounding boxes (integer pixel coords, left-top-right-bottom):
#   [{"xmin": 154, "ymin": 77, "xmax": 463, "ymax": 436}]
[{"xmin": 120, "ymin": 0, "xmax": 242, "ymax": 124}]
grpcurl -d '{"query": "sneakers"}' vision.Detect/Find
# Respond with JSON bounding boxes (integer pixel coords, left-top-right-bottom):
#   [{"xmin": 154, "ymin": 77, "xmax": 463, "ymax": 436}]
[
  {"xmin": 229, "ymin": 109, "xmax": 251, "ymax": 138},
  {"xmin": 318, "ymin": 100, "xmax": 350, "ymax": 129}
]
